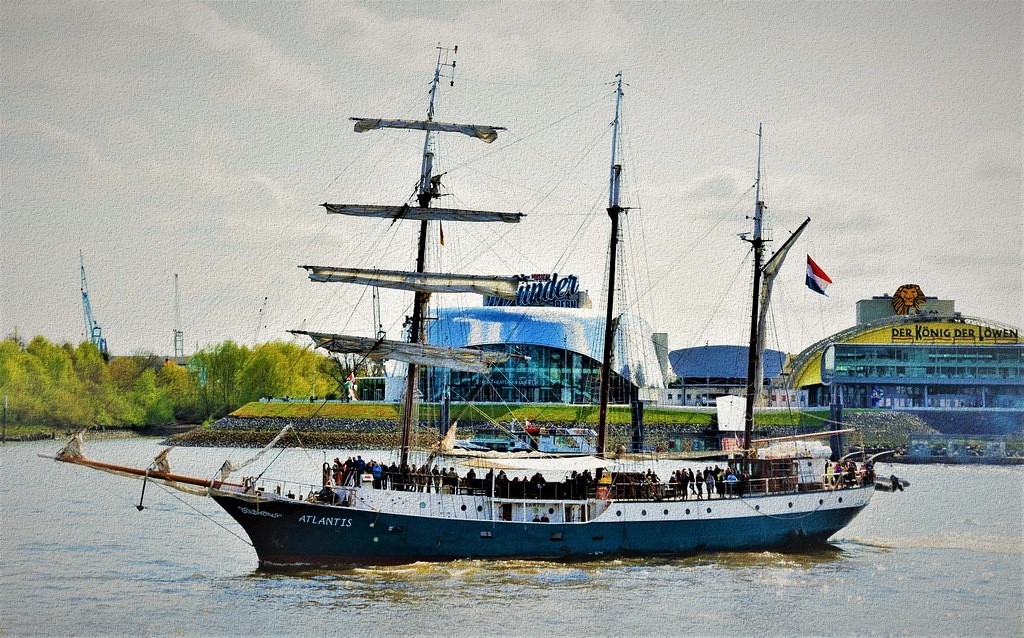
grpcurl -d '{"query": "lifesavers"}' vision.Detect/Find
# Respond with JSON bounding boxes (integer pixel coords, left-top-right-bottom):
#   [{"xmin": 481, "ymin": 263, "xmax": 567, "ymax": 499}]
[
  {"xmin": 596, "ymin": 488, "xmax": 610, "ymax": 500},
  {"xmin": 859, "ymin": 466, "xmax": 867, "ymax": 478}
]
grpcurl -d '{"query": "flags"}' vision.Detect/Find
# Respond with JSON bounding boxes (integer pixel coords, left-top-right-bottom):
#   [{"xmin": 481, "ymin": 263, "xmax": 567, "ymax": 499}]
[{"xmin": 804, "ymin": 254, "xmax": 831, "ymax": 297}]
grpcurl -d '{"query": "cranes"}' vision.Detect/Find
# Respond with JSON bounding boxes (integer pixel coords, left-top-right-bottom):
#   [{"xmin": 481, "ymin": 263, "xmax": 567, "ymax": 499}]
[
  {"xmin": 168, "ymin": 274, "xmax": 186, "ymax": 366},
  {"xmin": 76, "ymin": 247, "xmax": 110, "ymax": 362}
]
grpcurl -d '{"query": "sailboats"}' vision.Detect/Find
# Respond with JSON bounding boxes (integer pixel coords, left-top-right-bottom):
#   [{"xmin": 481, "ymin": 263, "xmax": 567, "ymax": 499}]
[{"xmin": 35, "ymin": 38, "xmax": 915, "ymax": 574}]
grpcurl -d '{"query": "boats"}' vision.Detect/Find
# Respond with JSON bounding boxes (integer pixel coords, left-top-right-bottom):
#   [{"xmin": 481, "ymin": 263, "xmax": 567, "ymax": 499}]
[{"xmin": 891, "ymin": 433, "xmax": 1024, "ymax": 466}]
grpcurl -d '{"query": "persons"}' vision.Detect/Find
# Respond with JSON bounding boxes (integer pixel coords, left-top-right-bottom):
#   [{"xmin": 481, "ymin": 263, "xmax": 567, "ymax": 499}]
[{"xmin": 330, "ymin": 455, "xmax": 877, "ymax": 500}]
